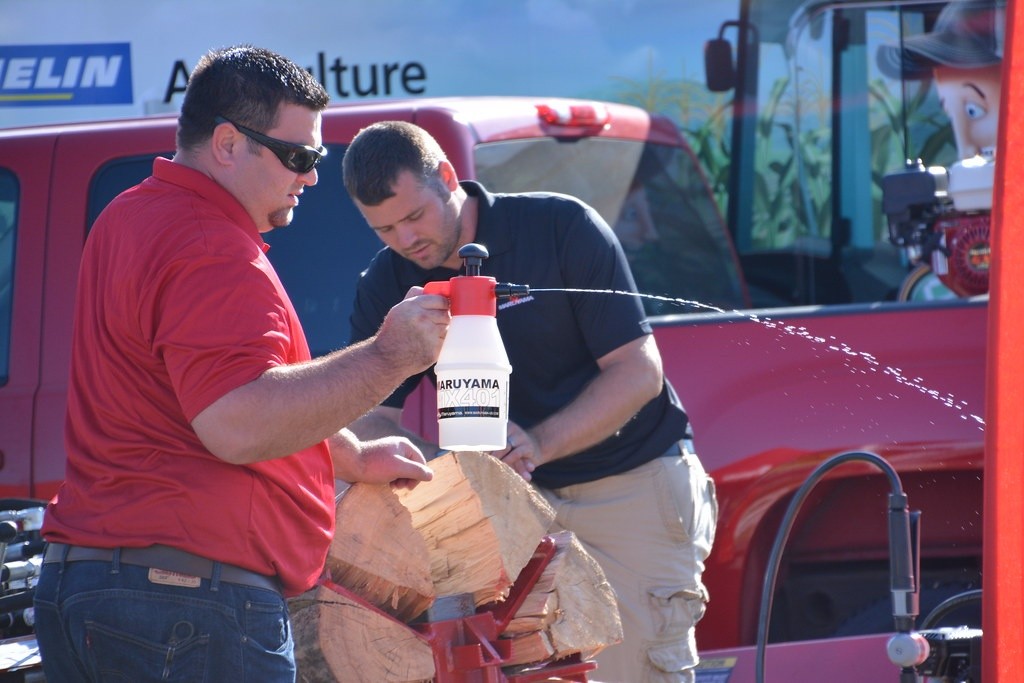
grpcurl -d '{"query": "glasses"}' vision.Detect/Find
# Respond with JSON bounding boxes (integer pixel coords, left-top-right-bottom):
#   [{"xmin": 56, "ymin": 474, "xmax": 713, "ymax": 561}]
[{"xmin": 210, "ymin": 114, "xmax": 326, "ymax": 174}]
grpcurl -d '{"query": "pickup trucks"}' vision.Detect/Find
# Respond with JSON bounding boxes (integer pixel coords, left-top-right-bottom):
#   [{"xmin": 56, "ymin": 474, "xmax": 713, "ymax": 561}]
[{"xmin": 1, "ymin": 96, "xmax": 984, "ymax": 682}]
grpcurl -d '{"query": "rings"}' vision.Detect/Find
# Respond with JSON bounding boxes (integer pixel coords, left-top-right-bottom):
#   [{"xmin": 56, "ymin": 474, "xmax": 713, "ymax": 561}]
[{"xmin": 507, "ymin": 437, "xmax": 516, "ymax": 449}]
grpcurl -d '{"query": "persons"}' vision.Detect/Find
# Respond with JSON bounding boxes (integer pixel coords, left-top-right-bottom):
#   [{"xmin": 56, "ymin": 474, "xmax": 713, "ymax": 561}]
[
  {"xmin": 342, "ymin": 118, "xmax": 718, "ymax": 683},
  {"xmin": 32, "ymin": 48, "xmax": 450, "ymax": 682}
]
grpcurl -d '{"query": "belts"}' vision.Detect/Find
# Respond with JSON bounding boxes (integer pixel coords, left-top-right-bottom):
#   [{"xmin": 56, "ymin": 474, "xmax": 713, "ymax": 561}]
[
  {"xmin": 662, "ymin": 438, "xmax": 695, "ymax": 456},
  {"xmin": 43, "ymin": 542, "xmax": 280, "ymax": 592}
]
